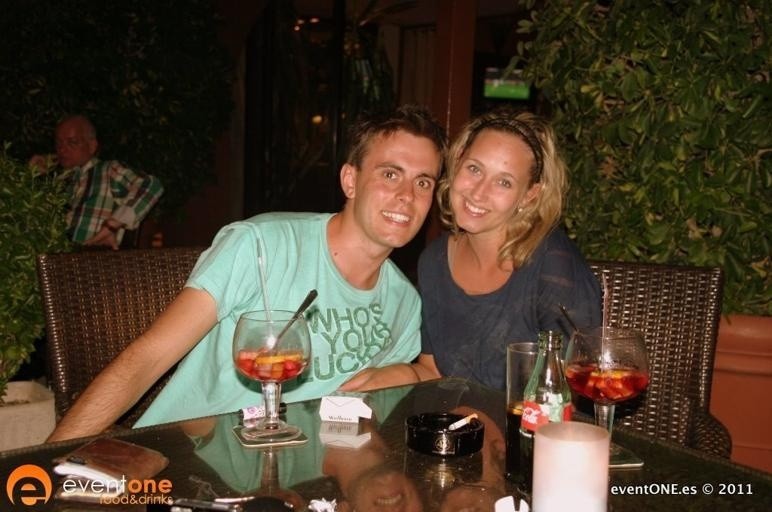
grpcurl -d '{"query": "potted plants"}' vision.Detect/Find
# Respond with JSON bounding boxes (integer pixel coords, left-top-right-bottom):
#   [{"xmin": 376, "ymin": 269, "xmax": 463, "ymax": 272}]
[
  {"xmin": 505, "ymin": 1, "xmax": 771, "ymax": 473},
  {"xmin": 0, "ymin": 142, "xmax": 82, "ymax": 452},
  {"xmin": 9, "ymin": 6, "xmax": 234, "ymax": 249}
]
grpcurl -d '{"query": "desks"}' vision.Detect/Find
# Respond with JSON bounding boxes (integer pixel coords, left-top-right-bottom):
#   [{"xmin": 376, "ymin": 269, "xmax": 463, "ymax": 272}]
[{"xmin": 1, "ymin": 377, "xmax": 772, "ymax": 512}]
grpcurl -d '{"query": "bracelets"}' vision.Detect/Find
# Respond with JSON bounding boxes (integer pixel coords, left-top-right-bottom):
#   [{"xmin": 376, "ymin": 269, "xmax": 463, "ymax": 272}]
[{"xmin": 102, "ymin": 221, "xmax": 117, "ymax": 233}]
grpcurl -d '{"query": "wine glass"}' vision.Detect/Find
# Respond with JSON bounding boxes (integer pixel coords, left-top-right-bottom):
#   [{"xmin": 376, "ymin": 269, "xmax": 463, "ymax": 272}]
[
  {"xmin": 232, "ymin": 310, "xmax": 313, "ymax": 442},
  {"xmin": 563, "ymin": 326, "xmax": 649, "ymax": 456}
]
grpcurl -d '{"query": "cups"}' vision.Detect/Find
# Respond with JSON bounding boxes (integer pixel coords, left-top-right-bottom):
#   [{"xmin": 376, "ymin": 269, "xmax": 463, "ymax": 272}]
[
  {"xmin": 504, "ymin": 340, "xmax": 563, "ymax": 483},
  {"xmin": 531, "ymin": 421, "xmax": 611, "ymax": 510}
]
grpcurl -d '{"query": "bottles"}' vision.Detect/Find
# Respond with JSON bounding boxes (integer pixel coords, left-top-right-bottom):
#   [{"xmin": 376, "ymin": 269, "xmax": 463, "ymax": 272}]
[{"xmin": 520, "ymin": 331, "xmax": 572, "ymax": 498}]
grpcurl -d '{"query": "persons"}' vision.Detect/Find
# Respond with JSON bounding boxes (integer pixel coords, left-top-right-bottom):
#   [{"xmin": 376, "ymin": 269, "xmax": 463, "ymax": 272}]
[
  {"xmin": 177, "ymin": 380, "xmax": 513, "ymax": 512},
  {"xmin": 337, "ymin": 110, "xmax": 603, "ymax": 394},
  {"xmin": 29, "ymin": 115, "xmax": 166, "ymax": 250},
  {"xmin": 46, "ymin": 103, "xmax": 450, "ymax": 444}
]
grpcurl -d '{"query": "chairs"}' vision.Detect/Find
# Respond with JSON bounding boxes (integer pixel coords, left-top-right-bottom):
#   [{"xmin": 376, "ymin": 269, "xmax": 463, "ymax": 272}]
[
  {"xmin": 38, "ymin": 248, "xmax": 207, "ymax": 434},
  {"xmin": 583, "ymin": 259, "xmax": 732, "ymax": 460}
]
